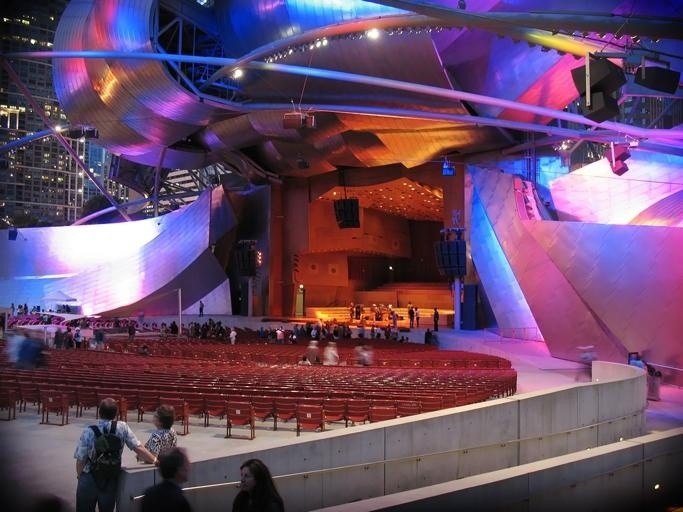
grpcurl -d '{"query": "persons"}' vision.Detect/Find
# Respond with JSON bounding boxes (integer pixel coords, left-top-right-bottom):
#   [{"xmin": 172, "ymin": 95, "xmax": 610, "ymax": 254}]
[
  {"xmin": 232, "ymin": 459, "xmax": 284, "ymax": 512},
  {"xmin": 73, "ymin": 398, "xmax": 194, "ymax": 512}
]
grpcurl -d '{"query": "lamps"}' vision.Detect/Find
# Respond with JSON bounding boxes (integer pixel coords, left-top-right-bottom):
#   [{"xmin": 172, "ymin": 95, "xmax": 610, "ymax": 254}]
[{"xmin": 571, "ymin": 55, "xmax": 680, "ymax": 124}]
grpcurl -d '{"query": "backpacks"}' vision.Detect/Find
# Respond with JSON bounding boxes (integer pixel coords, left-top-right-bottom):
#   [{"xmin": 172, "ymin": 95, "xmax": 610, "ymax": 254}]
[{"xmin": 88, "ymin": 421, "xmax": 122, "ymax": 492}]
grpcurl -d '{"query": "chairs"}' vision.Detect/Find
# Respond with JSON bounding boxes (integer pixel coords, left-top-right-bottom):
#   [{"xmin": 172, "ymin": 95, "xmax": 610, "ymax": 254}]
[{"xmin": 0, "ymin": 366, "xmax": 517, "ymax": 438}]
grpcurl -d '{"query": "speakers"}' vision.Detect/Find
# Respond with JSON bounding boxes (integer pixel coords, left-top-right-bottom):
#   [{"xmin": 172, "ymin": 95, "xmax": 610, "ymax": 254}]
[{"xmin": 463, "ymin": 284, "xmax": 478, "ymax": 330}]
